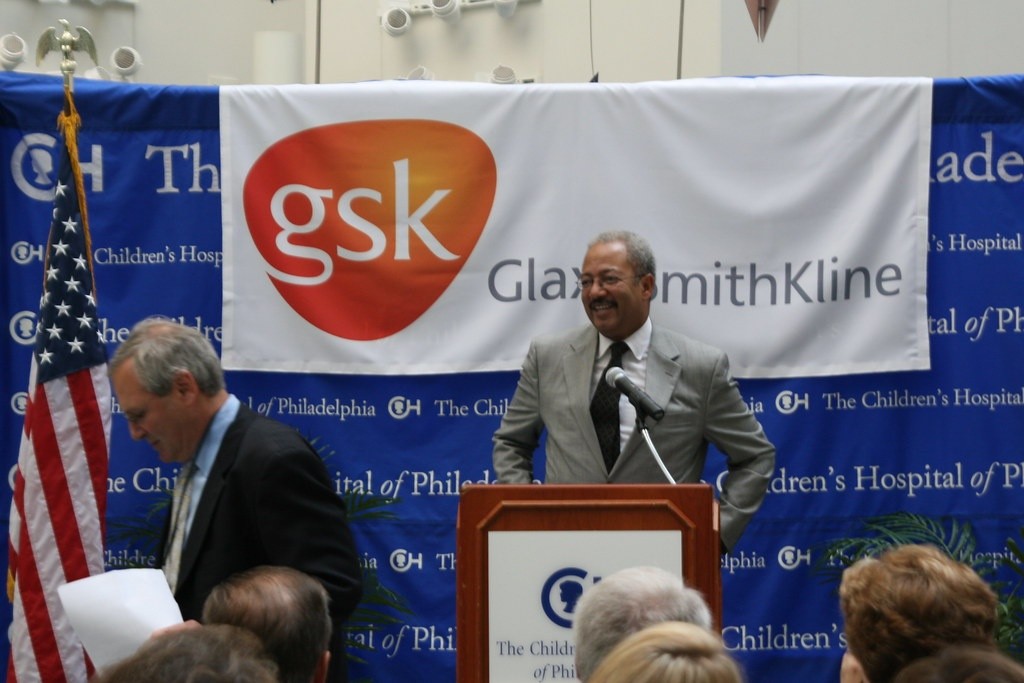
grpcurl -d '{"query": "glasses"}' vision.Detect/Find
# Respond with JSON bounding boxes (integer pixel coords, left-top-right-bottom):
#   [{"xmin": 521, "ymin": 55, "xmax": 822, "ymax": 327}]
[
  {"xmin": 574, "ymin": 274, "xmax": 644, "ymax": 292},
  {"xmin": 121, "ymin": 392, "xmax": 159, "ymax": 424}
]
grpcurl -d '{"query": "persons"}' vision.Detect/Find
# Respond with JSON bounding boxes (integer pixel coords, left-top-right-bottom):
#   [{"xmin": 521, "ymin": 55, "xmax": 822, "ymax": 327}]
[
  {"xmin": 571, "ymin": 568, "xmax": 743, "ymax": 683},
  {"xmin": 490, "ymin": 228, "xmax": 775, "ymax": 558},
  {"xmin": 840, "ymin": 543, "xmax": 1024, "ymax": 683},
  {"xmin": 91, "ymin": 563, "xmax": 331, "ymax": 683},
  {"xmin": 105, "ymin": 316, "xmax": 363, "ymax": 683}
]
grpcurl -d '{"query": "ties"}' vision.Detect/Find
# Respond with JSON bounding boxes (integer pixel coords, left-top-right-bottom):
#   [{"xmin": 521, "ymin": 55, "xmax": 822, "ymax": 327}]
[
  {"xmin": 162, "ymin": 463, "xmax": 192, "ymax": 597},
  {"xmin": 589, "ymin": 341, "xmax": 630, "ymax": 476}
]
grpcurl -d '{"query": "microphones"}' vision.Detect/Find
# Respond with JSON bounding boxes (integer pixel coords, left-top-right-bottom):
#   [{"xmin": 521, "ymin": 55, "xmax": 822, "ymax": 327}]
[{"xmin": 605, "ymin": 367, "xmax": 664, "ymax": 422}]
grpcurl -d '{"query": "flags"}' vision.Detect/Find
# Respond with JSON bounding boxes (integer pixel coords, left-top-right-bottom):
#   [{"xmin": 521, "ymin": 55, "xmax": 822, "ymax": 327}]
[{"xmin": 6, "ymin": 99, "xmax": 113, "ymax": 682}]
